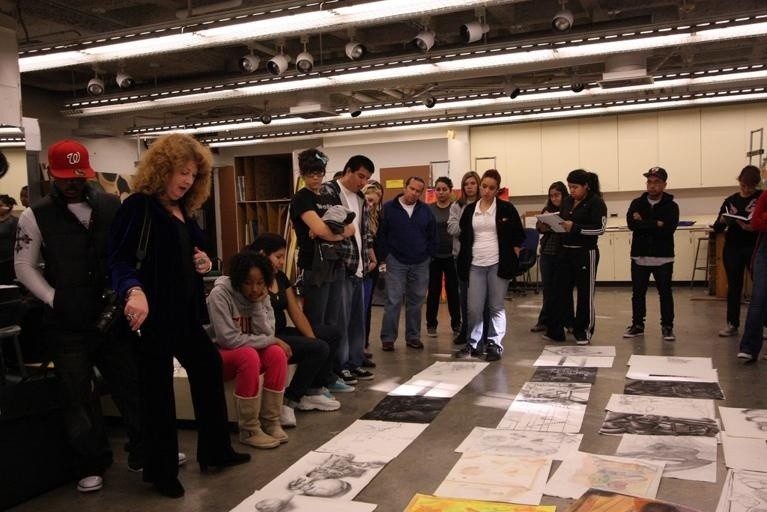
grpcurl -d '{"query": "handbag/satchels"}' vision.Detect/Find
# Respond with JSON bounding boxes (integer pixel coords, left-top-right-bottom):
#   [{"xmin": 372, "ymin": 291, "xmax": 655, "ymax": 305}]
[{"xmin": 85, "ymin": 286, "xmax": 145, "ymax": 359}]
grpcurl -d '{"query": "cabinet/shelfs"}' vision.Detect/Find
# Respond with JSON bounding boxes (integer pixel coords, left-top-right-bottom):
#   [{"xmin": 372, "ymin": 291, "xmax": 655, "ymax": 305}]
[
  {"xmin": 467, "ymin": 117, "xmax": 766, "ymax": 200},
  {"xmin": 505, "ymin": 216, "xmax": 719, "ymax": 286}
]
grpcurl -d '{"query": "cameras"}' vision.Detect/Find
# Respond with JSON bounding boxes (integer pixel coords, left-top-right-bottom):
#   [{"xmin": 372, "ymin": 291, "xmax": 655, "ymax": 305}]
[{"xmin": 89, "ymin": 289, "xmax": 124, "ymax": 342}]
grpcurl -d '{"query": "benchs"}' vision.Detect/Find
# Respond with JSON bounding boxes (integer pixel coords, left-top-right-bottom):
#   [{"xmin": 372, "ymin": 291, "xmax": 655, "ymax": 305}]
[{"xmin": 96, "ymin": 361, "xmax": 302, "ymax": 428}]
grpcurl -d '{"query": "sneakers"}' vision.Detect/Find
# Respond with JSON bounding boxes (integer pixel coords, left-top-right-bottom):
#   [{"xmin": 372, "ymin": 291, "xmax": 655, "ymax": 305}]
[
  {"xmin": 282, "ymin": 406, "xmax": 296, "ymax": 425},
  {"xmin": 719, "ymin": 324, "xmax": 739, "ymax": 336},
  {"xmin": 127, "ymin": 451, "xmax": 187, "ymax": 498},
  {"xmin": 383, "ymin": 342, "xmax": 394, "ymax": 350},
  {"xmin": 406, "ymin": 339, "xmax": 424, "ymax": 349},
  {"xmin": 736, "ymin": 351, "xmax": 755, "ymax": 359},
  {"xmin": 661, "ymin": 328, "xmax": 676, "ymax": 341},
  {"xmin": 426, "ymin": 328, "xmax": 437, "ymax": 335},
  {"xmin": 530, "ymin": 323, "xmax": 590, "ymax": 344},
  {"xmin": 78, "ymin": 464, "xmax": 104, "ymax": 492},
  {"xmin": 197, "ymin": 450, "xmax": 251, "ymax": 466},
  {"xmin": 298, "ymin": 358, "xmax": 375, "ymax": 411},
  {"xmin": 624, "ymin": 324, "xmax": 645, "ymax": 338},
  {"xmin": 453, "ymin": 326, "xmax": 502, "ymax": 361}
]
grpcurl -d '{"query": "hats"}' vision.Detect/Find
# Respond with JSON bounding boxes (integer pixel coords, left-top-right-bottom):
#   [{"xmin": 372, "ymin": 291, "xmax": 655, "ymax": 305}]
[
  {"xmin": 48, "ymin": 140, "xmax": 96, "ymax": 179},
  {"xmin": 643, "ymin": 167, "xmax": 668, "ymax": 182}
]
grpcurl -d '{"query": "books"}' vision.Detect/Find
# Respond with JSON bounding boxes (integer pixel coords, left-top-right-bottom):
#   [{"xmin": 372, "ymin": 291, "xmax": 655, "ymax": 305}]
[
  {"xmin": 534, "ymin": 213, "xmax": 570, "ymax": 233},
  {"xmin": 722, "ymin": 212, "xmax": 752, "ymax": 223}
]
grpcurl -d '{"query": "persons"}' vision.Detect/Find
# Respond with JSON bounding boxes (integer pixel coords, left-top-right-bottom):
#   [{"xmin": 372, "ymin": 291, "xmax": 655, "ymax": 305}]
[
  {"xmin": 371, "ymin": 169, "xmax": 525, "ymax": 362},
  {"xmin": 211, "ymin": 235, "xmax": 343, "ymax": 447},
  {"xmin": 737, "ymin": 188, "xmax": 767, "ymax": 362},
  {"xmin": 624, "ymin": 166, "xmax": 679, "ymax": 341},
  {"xmin": 542, "ymin": 169, "xmax": 607, "ymax": 345},
  {"xmin": 291, "ymin": 149, "xmax": 384, "ymax": 399},
  {"xmin": 713, "ymin": 164, "xmax": 763, "ymax": 336},
  {"xmin": 3, "ymin": 135, "xmax": 251, "ymax": 498},
  {"xmin": 530, "ymin": 181, "xmax": 571, "ymax": 331}
]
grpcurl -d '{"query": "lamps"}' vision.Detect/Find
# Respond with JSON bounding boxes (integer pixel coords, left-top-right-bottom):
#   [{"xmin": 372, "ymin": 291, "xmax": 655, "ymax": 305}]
[
  {"xmin": 570, "ymin": 67, "xmax": 585, "ymax": 93},
  {"xmin": 423, "ymin": 84, "xmax": 438, "ymax": 107},
  {"xmin": 240, "ymin": 40, "xmax": 261, "ymax": 74},
  {"xmin": 295, "ymin": 33, "xmax": 314, "ymax": 74},
  {"xmin": 268, "ymin": 41, "xmax": 293, "ymax": 75},
  {"xmin": 348, "ymin": 95, "xmax": 362, "ymax": 118},
  {"xmin": 504, "ymin": 76, "xmax": 521, "ymax": 99},
  {"xmin": 344, "ymin": 27, "xmax": 363, "ymax": 61},
  {"xmin": 461, "ymin": 6, "xmax": 489, "ymax": 45},
  {"xmin": 86, "ymin": 69, "xmax": 104, "ymax": 94},
  {"xmin": 553, "ymin": 2, "xmax": 574, "ymax": 32},
  {"xmin": 260, "ymin": 100, "xmax": 271, "ymax": 124},
  {"xmin": 114, "ymin": 69, "xmax": 134, "ymax": 89},
  {"xmin": 411, "ymin": 16, "xmax": 437, "ymax": 53}
]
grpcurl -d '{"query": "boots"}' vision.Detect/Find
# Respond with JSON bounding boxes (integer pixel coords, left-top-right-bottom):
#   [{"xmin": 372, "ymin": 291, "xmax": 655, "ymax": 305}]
[{"xmin": 231, "ymin": 387, "xmax": 289, "ymax": 450}]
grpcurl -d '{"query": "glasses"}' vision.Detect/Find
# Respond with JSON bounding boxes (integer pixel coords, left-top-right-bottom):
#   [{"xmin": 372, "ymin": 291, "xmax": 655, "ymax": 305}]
[{"xmin": 305, "ymin": 170, "xmax": 326, "ymax": 177}]
